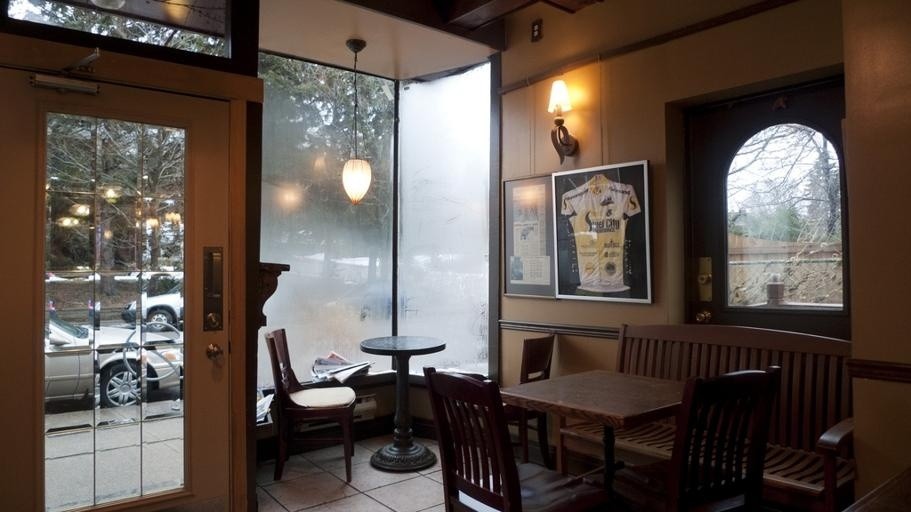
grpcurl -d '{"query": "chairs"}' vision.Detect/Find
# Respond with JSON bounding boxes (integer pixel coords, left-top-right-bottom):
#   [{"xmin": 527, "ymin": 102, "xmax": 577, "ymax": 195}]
[
  {"xmin": 265, "ymin": 328, "xmax": 358, "ymax": 485},
  {"xmin": 457, "ymin": 334, "xmax": 557, "ymax": 460},
  {"xmin": 422, "ymin": 367, "xmax": 603, "ymax": 512},
  {"xmin": 610, "ymin": 365, "xmax": 782, "ymax": 511}
]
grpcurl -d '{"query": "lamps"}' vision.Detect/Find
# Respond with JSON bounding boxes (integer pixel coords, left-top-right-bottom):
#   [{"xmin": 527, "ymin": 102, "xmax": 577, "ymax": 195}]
[
  {"xmin": 340, "ymin": 39, "xmax": 374, "ymax": 208},
  {"xmin": 548, "ymin": 77, "xmax": 578, "ymax": 166}
]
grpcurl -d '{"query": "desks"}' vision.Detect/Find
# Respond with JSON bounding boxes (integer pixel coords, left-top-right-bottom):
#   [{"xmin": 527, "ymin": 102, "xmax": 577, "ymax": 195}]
[
  {"xmin": 488, "ymin": 369, "xmax": 687, "ymax": 511},
  {"xmin": 360, "ymin": 336, "xmax": 446, "ymax": 472}
]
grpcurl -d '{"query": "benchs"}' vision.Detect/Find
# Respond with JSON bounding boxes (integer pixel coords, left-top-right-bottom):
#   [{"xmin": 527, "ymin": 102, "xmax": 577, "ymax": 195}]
[{"xmin": 559, "ymin": 322, "xmax": 853, "ymax": 512}]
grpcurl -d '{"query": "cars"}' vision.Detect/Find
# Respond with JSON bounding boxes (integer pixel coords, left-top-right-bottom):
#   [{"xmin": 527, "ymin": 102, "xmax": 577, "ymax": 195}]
[
  {"xmin": 123, "ymin": 276, "xmax": 184, "ymax": 332},
  {"xmin": 257, "ymin": 268, "xmax": 489, "ymax": 369},
  {"xmin": 44, "ymin": 308, "xmax": 185, "ymax": 408}
]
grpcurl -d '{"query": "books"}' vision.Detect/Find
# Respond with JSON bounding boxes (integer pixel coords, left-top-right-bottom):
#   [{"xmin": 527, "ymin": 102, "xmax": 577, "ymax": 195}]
[
  {"xmin": 308, "ymin": 352, "xmax": 375, "ymax": 384},
  {"xmin": 256, "ymin": 393, "xmax": 274, "ymax": 424}
]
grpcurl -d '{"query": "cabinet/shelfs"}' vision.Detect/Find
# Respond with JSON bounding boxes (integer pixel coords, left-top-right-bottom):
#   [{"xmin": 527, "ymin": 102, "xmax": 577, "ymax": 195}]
[{"xmin": 258, "ymin": 259, "xmax": 290, "ymax": 327}]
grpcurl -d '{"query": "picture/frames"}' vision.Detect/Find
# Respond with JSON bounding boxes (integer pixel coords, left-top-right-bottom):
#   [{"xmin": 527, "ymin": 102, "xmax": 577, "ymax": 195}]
[
  {"xmin": 501, "ymin": 172, "xmax": 558, "ymax": 300},
  {"xmin": 550, "ymin": 158, "xmax": 654, "ymax": 305}
]
